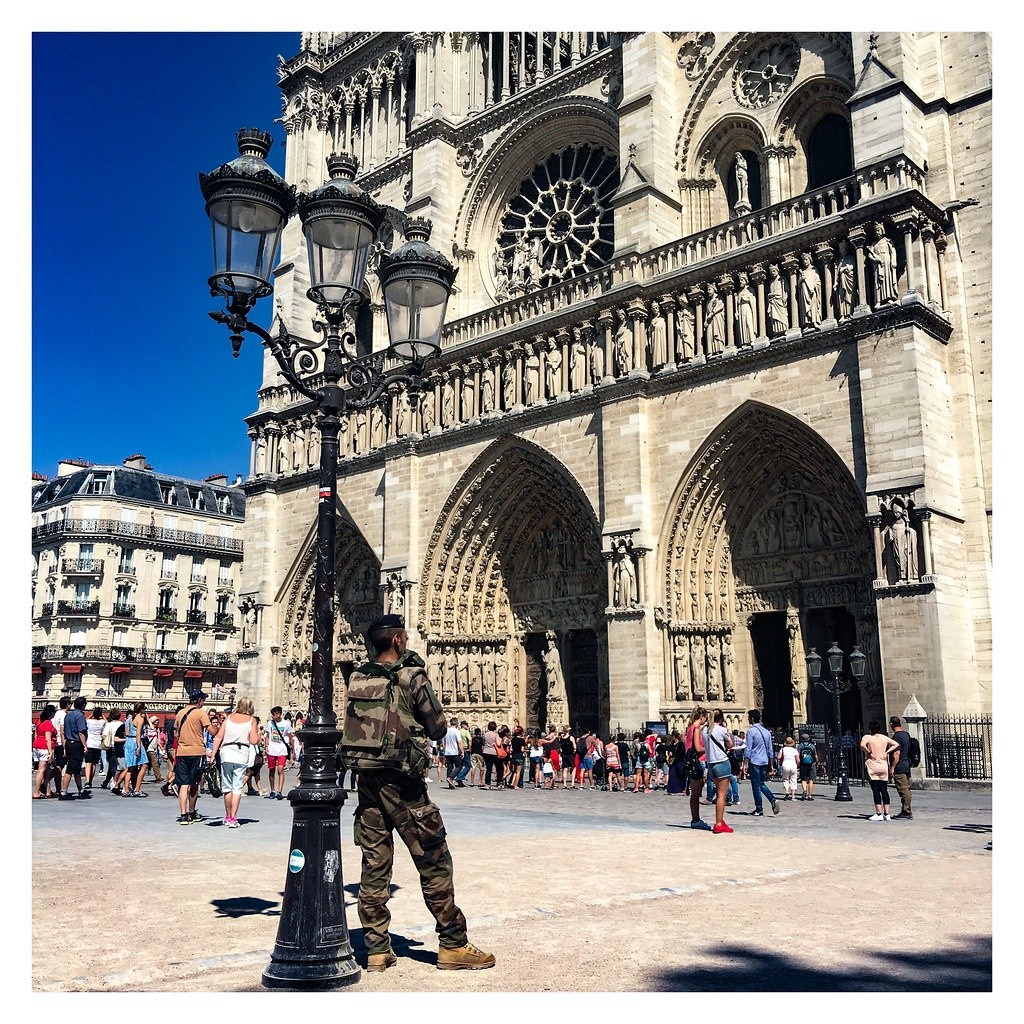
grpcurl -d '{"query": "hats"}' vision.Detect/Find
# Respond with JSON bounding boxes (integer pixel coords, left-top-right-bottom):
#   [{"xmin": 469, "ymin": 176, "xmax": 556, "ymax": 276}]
[
  {"xmin": 367, "ymin": 613, "xmax": 406, "ymax": 632},
  {"xmin": 190, "ymin": 688, "xmax": 208, "ymax": 701},
  {"xmin": 801, "ymin": 734, "xmax": 810, "ymax": 741},
  {"xmin": 783, "ymin": 737, "xmax": 795, "ymax": 747},
  {"xmin": 149, "ymin": 715, "xmax": 160, "ymax": 724}
]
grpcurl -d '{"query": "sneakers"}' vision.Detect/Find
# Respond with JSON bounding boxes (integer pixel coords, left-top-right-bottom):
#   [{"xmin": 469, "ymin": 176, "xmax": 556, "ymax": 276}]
[
  {"xmin": 33, "ymin": 774, "xmax": 283, "ymax": 829},
  {"xmin": 367, "ymin": 949, "xmax": 398, "ymax": 971},
  {"xmin": 422, "ymin": 774, "xmax": 914, "ymax": 834},
  {"xmin": 438, "ymin": 942, "xmax": 495, "ymax": 972}
]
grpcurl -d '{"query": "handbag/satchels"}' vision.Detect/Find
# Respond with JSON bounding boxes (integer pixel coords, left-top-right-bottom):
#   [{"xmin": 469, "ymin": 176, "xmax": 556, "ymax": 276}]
[
  {"xmin": 287, "ymin": 747, "xmax": 294, "ymax": 761},
  {"xmin": 893, "ymin": 731, "xmax": 921, "ymax": 767},
  {"xmin": 728, "ymin": 752, "xmax": 740, "ymax": 776},
  {"xmin": 767, "ymin": 760, "xmax": 777, "ymax": 772},
  {"xmin": 101, "ymin": 732, "xmax": 113, "ymax": 750},
  {"xmin": 684, "ymin": 727, "xmax": 703, "ymax": 781}
]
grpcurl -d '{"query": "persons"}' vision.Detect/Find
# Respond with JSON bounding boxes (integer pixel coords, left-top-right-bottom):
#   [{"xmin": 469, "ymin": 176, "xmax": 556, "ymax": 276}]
[
  {"xmin": 389, "ymin": 581, "xmax": 402, "ymax": 614},
  {"xmin": 676, "ymin": 635, "xmax": 735, "ymax": 692},
  {"xmin": 256, "ymin": 221, "xmax": 899, "ymax": 478},
  {"xmin": 686, "ymin": 705, "xmax": 734, "ymax": 833},
  {"xmin": 288, "ymin": 670, "xmax": 309, "ymax": 704},
  {"xmin": 428, "ymin": 645, "xmax": 507, "ymax": 695},
  {"xmin": 541, "ymin": 641, "xmax": 560, "ymax": 696},
  {"xmin": 735, "ymin": 152, "xmax": 751, "ymax": 203},
  {"xmin": 613, "ymin": 546, "xmax": 637, "ymax": 608},
  {"xmin": 888, "ymin": 505, "xmax": 918, "ymax": 582},
  {"xmin": 32, "ymin": 690, "xmax": 304, "ymax": 828},
  {"xmin": 778, "ymin": 734, "xmax": 818, "ymax": 800},
  {"xmin": 425, "ymin": 717, "xmax": 690, "ymax": 795},
  {"xmin": 742, "ymin": 709, "xmax": 780, "ymax": 815},
  {"xmin": 339, "ymin": 771, "xmax": 358, "ymax": 793},
  {"xmin": 860, "ymin": 716, "xmax": 913, "ymax": 820},
  {"xmin": 354, "ymin": 614, "xmax": 496, "ymax": 972},
  {"xmin": 246, "ymin": 609, "xmax": 256, "ymax": 649},
  {"xmin": 700, "ymin": 721, "xmax": 749, "ymax": 805}
]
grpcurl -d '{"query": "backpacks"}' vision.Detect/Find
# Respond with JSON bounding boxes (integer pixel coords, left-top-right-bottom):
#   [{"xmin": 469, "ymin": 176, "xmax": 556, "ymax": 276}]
[
  {"xmin": 800, "ymin": 742, "xmax": 814, "ymax": 765},
  {"xmin": 495, "ymin": 742, "xmax": 508, "ymax": 758},
  {"xmin": 577, "ymin": 736, "xmax": 592, "ymax": 758},
  {"xmin": 639, "ymin": 743, "xmax": 649, "ymax": 763},
  {"xmin": 338, "ymin": 661, "xmax": 412, "ymax": 791}
]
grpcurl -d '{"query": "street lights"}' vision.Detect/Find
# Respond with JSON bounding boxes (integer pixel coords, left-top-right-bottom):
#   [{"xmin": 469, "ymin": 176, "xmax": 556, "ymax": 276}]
[
  {"xmin": 805, "ymin": 643, "xmax": 870, "ymax": 802},
  {"xmin": 228, "ymin": 687, "xmax": 237, "ymax": 713},
  {"xmin": 200, "ymin": 132, "xmax": 460, "ymax": 992}
]
